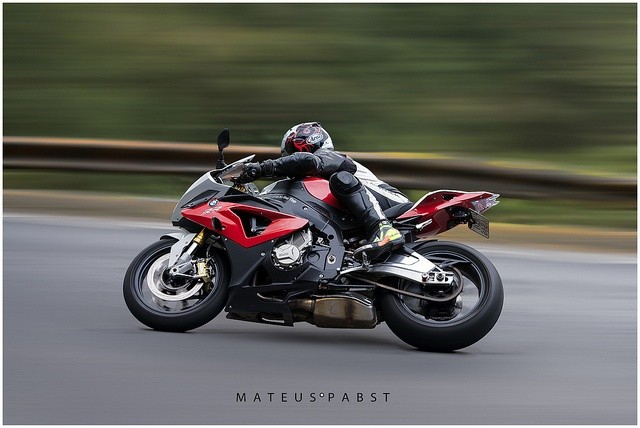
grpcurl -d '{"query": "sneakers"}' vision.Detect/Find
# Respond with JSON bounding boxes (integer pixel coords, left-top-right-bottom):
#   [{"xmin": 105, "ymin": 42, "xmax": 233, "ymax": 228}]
[{"xmin": 355, "ymin": 220, "xmax": 406, "ymax": 263}]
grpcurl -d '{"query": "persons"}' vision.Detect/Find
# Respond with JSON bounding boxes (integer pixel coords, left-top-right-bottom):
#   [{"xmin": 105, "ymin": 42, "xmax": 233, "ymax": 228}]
[{"xmin": 240, "ymin": 121, "xmax": 416, "ymax": 259}]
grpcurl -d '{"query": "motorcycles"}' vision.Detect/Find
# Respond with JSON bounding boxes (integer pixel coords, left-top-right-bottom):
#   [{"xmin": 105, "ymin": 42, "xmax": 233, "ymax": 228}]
[{"xmin": 122, "ymin": 127, "xmax": 504, "ymax": 352}]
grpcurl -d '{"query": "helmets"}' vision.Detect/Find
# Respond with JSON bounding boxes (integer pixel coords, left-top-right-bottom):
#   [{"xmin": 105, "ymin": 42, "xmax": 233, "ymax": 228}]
[{"xmin": 281, "ymin": 122, "xmax": 334, "ymax": 157}]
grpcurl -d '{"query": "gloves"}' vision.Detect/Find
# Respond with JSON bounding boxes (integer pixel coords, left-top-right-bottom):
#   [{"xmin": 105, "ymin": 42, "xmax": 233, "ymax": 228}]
[{"xmin": 237, "ymin": 163, "xmax": 261, "ymax": 183}]
